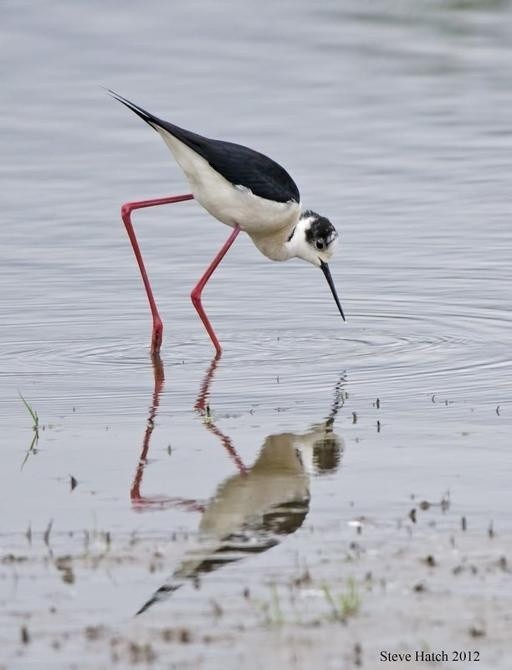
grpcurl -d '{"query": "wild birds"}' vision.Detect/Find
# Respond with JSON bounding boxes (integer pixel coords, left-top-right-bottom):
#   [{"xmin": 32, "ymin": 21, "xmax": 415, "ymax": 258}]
[
  {"xmin": 111, "ymin": 362, "xmax": 348, "ymax": 618},
  {"xmin": 94, "ymin": 84, "xmax": 345, "ymax": 363}
]
